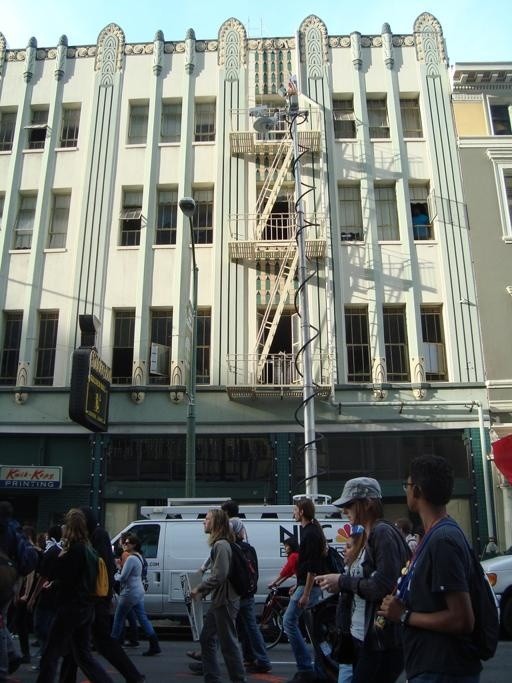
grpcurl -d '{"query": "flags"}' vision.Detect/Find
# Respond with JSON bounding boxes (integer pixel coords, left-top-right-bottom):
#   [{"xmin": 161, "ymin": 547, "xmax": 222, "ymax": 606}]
[{"xmin": 489, "ymin": 430, "xmax": 512, "ymax": 487}]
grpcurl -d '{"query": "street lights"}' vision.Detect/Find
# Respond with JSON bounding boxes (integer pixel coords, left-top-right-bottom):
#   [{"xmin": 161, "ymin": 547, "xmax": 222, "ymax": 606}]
[{"xmin": 176, "ymin": 193, "xmax": 210, "ymax": 496}]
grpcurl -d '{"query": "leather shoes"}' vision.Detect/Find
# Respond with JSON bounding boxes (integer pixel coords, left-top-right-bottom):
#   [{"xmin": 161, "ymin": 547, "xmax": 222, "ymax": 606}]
[
  {"xmin": 246, "ymin": 664, "xmax": 272, "ymax": 673},
  {"xmin": 186, "ymin": 650, "xmax": 200, "ymax": 660},
  {"xmin": 188, "ymin": 663, "xmax": 201, "ymax": 671}
]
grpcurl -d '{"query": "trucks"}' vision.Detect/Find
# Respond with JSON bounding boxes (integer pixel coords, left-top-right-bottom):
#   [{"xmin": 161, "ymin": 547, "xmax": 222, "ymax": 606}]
[{"xmin": 477, "ymin": 544, "xmax": 511, "ymax": 638}]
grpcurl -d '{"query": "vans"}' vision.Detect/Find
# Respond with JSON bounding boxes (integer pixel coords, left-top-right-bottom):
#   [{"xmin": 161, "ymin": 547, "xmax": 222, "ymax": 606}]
[{"xmin": 102, "ymin": 495, "xmax": 353, "ymax": 640}]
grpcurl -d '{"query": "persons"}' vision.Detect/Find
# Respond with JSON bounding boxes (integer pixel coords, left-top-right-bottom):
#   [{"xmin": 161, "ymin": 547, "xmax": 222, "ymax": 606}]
[
  {"xmin": 186, "ymin": 499, "xmax": 273, "ymax": 681},
  {"xmin": 312, "ymin": 476, "xmax": 413, "ymax": 682},
  {"xmin": 0, "ymin": 496, "xmax": 162, "ymax": 683},
  {"xmin": 338, "ymin": 533, "xmax": 365, "ymax": 565},
  {"xmin": 283, "ymin": 497, "xmax": 332, "ymax": 682},
  {"xmin": 412, "ymin": 526, "xmax": 424, "ymax": 544},
  {"xmin": 374, "ymin": 453, "xmax": 484, "ymax": 682},
  {"xmin": 269, "ymin": 535, "xmax": 316, "ymax": 644},
  {"xmin": 393, "ymin": 518, "xmax": 418, "ymax": 553}
]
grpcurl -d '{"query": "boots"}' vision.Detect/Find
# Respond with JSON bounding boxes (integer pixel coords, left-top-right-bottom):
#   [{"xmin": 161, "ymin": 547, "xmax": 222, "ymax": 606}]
[{"xmin": 143, "ymin": 633, "xmax": 161, "ymax": 655}]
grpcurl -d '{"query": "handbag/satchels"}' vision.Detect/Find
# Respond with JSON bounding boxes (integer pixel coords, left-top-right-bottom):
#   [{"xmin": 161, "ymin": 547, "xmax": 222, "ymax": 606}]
[
  {"xmin": 325, "ymin": 545, "xmax": 344, "ymax": 573},
  {"xmin": 95, "ymin": 556, "xmax": 109, "ymax": 596}
]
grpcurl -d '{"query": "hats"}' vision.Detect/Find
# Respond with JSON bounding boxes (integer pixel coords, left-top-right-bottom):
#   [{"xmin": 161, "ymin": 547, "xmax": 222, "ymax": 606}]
[
  {"xmin": 332, "ymin": 476, "xmax": 382, "ymax": 508},
  {"xmin": 229, "ymin": 516, "xmax": 243, "ymax": 533}
]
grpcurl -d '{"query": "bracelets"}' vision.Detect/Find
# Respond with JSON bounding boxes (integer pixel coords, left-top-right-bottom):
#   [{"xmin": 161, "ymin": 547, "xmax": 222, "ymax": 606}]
[{"xmin": 400, "ymin": 609, "xmax": 411, "ymax": 626}]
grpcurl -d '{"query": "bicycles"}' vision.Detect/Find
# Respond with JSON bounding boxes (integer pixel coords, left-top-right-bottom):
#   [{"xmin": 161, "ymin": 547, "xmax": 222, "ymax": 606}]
[{"xmin": 254, "ymin": 583, "xmax": 341, "ymax": 648}]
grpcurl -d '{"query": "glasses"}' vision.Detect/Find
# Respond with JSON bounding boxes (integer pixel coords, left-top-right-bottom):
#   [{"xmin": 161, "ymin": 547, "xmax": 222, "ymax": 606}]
[{"xmin": 402, "ymin": 480, "xmax": 414, "ymax": 492}]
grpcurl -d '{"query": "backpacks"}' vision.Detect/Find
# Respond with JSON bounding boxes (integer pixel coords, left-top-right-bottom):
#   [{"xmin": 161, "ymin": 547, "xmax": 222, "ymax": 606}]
[
  {"xmin": 5, "ymin": 520, "xmax": 38, "ymax": 576},
  {"xmin": 400, "ymin": 521, "xmax": 500, "ymax": 661},
  {"xmin": 228, "ymin": 542, "xmax": 259, "ymax": 598}
]
